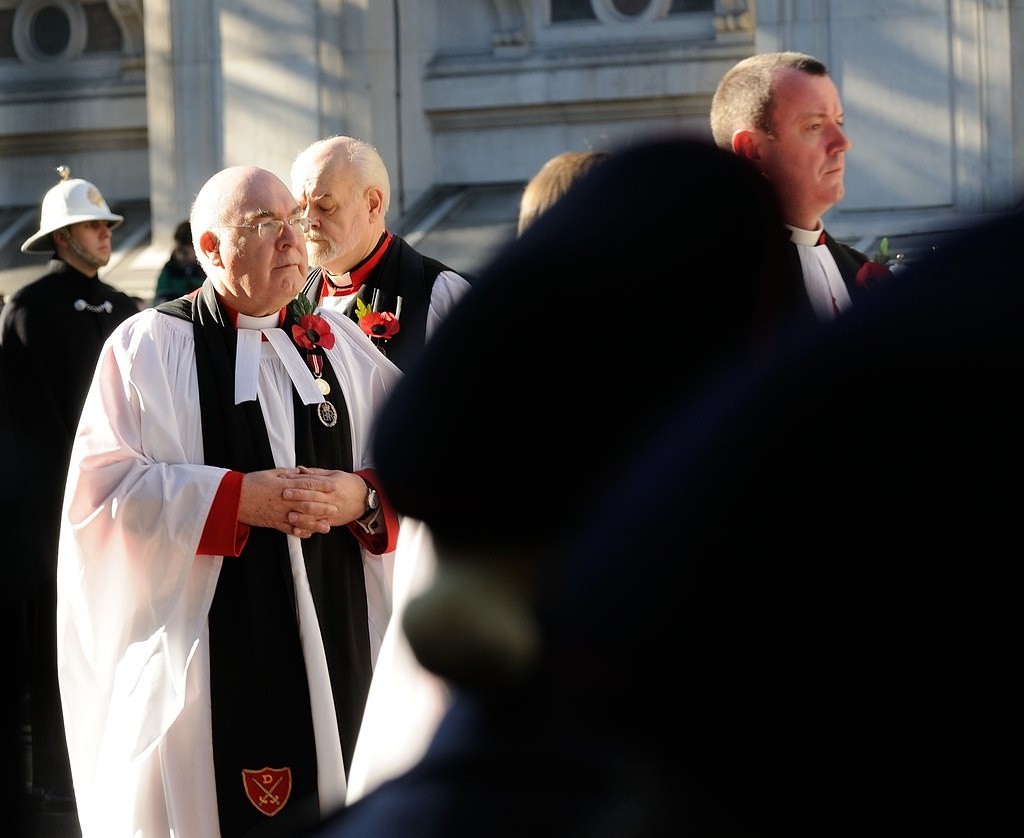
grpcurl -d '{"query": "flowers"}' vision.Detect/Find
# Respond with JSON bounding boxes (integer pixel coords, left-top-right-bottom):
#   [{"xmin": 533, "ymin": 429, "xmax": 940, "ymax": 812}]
[
  {"xmin": 856, "ymin": 236, "xmax": 894, "ymax": 284},
  {"xmin": 292, "ymin": 292, "xmax": 336, "ymax": 351},
  {"xmin": 353, "ymin": 295, "xmax": 399, "ymax": 339}
]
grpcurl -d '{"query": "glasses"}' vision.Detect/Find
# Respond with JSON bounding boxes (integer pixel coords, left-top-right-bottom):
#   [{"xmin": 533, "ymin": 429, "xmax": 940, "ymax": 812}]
[{"xmin": 206, "ymin": 218, "xmax": 311, "ymax": 239}]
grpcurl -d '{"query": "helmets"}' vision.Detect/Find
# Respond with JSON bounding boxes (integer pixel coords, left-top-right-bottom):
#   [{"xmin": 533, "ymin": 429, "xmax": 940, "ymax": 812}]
[{"xmin": 21, "ymin": 164, "xmax": 124, "ymax": 253}]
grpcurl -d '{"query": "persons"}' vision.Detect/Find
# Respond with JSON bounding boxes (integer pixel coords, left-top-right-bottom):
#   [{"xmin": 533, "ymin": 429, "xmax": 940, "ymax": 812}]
[{"xmin": 0, "ymin": 53, "xmax": 1024, "ymax": 838}]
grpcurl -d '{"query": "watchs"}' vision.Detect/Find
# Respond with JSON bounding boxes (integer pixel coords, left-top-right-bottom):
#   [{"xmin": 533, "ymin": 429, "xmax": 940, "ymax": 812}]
[{"xmin": 357, "ymin": 483, "xmax": 379, "ymax": 524}]
